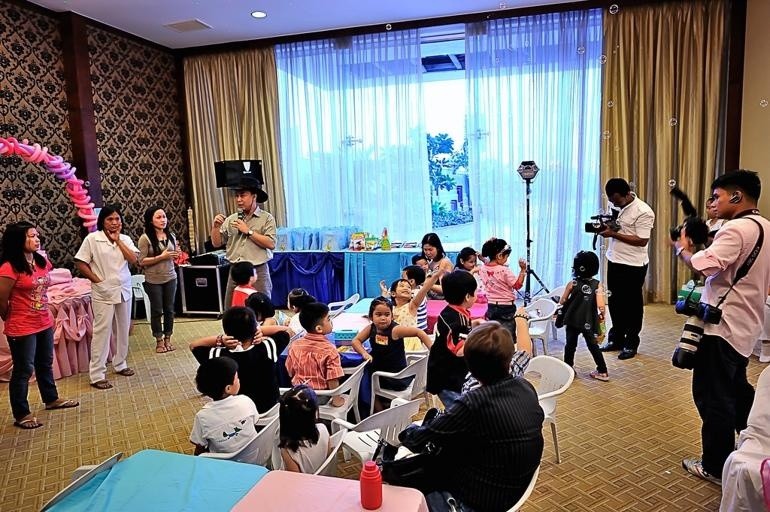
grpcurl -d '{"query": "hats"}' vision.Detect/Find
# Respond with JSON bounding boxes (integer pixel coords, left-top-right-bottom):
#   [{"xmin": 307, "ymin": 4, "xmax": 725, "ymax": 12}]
[
  {"xmin": 573, "ymin": 250, "xmax": 599, "ymax": 276},
  {"xmin": 229, "ymin": 176, "xmax": 268, "ymax": 205}
]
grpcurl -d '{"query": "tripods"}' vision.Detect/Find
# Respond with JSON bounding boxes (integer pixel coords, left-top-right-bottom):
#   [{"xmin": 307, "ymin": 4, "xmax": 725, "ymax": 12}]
[{"xmin": 517, "ymin": 182, "xmax": 558, "ymax": 307}]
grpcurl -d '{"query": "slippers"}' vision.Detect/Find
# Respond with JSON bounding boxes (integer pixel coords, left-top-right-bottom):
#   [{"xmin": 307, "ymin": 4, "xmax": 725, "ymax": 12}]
[
  {"xmin": 114, "ymin": 364, "xmax": 135, "ymax": 376},
  {"xmin": 89, "ymin": 376, "xmax": 113, "ymax": 391},
  {"xmin": 13, "ymin": 417, "xmax": 43, "ymax": 430},
  {"xmin": 45, "ymin": 398, "xmax": 80, "ymax": 410}
]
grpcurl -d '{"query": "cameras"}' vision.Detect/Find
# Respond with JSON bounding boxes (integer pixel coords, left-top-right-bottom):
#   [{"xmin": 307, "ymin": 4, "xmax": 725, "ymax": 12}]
[
  {"xmin": 670, "ymin": 187, "xmax": 709, "ymax": 245},
  {"xmin": 672, "ymin": 300, "xmax": 722, "ymax": 370}
]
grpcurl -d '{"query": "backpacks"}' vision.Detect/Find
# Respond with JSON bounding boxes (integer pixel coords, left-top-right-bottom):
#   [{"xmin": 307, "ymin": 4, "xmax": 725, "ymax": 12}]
[{"xmin": 556, "ymin": 277, "xmax": 597, "ymax": 328}]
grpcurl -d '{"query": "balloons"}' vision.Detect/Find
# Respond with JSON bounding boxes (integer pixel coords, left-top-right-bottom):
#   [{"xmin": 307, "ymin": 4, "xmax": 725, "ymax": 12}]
[{"xmin": 0, "ymin": 137, "xmax": 98, "ymax": 232}]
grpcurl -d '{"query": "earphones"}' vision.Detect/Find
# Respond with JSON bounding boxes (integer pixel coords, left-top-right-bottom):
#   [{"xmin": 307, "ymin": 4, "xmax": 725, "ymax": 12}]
[{"xmin": 730, "ymin": 195, "xmax": 738, "ymax": 203}]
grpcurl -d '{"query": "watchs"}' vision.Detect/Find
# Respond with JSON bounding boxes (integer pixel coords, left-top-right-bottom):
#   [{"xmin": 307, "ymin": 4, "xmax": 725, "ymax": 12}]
[
  {"xmin": 676, "ymin": 247, "xmax": 687, "ymax": 256},
  {"xmin": 246, "ymin": 230, "xmax": 253, "ymax": 236}
]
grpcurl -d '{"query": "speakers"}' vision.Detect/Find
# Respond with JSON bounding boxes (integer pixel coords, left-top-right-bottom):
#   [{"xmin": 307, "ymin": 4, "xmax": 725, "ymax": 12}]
[{"xmin": 214, "ymin": 160, "xmax": 264, "ymax": 188}]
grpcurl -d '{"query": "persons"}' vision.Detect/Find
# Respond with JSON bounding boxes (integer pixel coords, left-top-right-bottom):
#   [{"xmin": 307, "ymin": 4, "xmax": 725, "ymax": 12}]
[
  {"xmin": 0, "ymin": 222, "xmax": 79, "ymax": 429},
  {"xmin": 704, "ymin": 198, "xmax": 728, "ymax": 234},
  {"xmin": 190, "ymin": 262, "xmax": 344, "ymax": 475},
  {"xmin": 351, "ymin": 233, "xmax": 526, "ymax": 412},
  {"xmin": 74, "ymin": 205, "xmax": 140, "ymax": 389},
  {"xmin": 554, "ymin": 249, "xmax": 610, "ymax": 382},
  {"xmin": 398, "ymin": 320, "xmax": 545, "ymax": 512},
  {"xmin": 138, "ymin": 206, "xmax": 178, "ymax": 353},
  {"xmin": 598, "ymin": 179, "xmax": 655, "ymax": 359},
  {"xmin": 212, "ymin": 177, "xmax": 277, "ymax": 314},
  {"xmin": 671, "ymin": 170, "xmax": 770, "ymax": 487}
]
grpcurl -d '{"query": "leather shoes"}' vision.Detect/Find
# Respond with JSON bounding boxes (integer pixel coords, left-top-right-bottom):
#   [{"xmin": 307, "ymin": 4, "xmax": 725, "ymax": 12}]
[{"xmin": 599, "ymin": 340, "xmax": 638, "ymax": 360}]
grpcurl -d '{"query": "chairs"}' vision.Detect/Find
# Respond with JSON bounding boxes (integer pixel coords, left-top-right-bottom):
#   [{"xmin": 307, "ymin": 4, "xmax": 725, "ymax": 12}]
[{"xmin": 131, "ymin": 274, "xmax": 151, "ymax": 321}]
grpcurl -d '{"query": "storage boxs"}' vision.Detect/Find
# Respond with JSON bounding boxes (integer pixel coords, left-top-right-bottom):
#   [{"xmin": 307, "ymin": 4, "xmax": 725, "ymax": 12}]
[{"xmin": 176, "ymin": 263, "xmax": 231, "ymax": 315}]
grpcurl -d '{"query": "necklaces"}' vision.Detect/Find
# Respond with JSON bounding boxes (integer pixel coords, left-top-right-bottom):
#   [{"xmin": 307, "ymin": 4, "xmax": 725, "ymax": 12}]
[
  {"xmin": 27, "ymin": 259, "xmax": 37, "ymax": 272},
  {"xmin": 159, "ymin": 235, "xmax": 166, "ymax": 246}
]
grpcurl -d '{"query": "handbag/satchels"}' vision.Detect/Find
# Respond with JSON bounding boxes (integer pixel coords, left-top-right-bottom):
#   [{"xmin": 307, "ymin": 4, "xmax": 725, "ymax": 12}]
[{"xmin": 382, "ymin": 406, "xmax": 444, "ymax": 494}]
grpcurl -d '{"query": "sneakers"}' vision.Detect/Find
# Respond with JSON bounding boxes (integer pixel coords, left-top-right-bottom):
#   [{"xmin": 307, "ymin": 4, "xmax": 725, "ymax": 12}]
[
  {"xmin": 589, "ymin": 369, "xmax": 609, "ymax": 381},
  {"xmin": 680, "ymin": 455, "xmax": 724, "ymax": 486}
]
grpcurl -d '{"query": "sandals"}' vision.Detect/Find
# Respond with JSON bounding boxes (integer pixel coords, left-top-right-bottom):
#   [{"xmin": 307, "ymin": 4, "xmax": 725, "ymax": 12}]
[{"xmin": 154, "ymin": 336, "xmax": 176, "ymax": 353}]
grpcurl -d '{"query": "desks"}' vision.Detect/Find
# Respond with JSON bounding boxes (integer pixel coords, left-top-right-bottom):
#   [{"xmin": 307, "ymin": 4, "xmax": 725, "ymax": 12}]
[
  {"xmin": 267, "ymin": 243, "xmax": 470, "ymax": 306},
  {"xmin": 41, "ymin": 448, "xmax": 268, "ymax": 512},
  {"xmin": 0, "ymin": 277, "xmax": 117, "ymax": 383}
]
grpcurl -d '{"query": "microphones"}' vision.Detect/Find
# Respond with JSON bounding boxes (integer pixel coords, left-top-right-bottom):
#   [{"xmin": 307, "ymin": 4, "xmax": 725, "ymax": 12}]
[{"xmin": 236, "ymin": 207, "xmax": 243, "ymax": 235}]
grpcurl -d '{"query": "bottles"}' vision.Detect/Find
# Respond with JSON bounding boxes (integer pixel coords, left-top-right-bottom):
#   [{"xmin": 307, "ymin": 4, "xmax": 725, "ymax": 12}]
[
  {"xmin": 360, "ymin": 460, "xmax": 382, "ymax": 509},
  {"xmin": 381, "ymin": 229, "xmax": 392, "ymax": 250}
]
